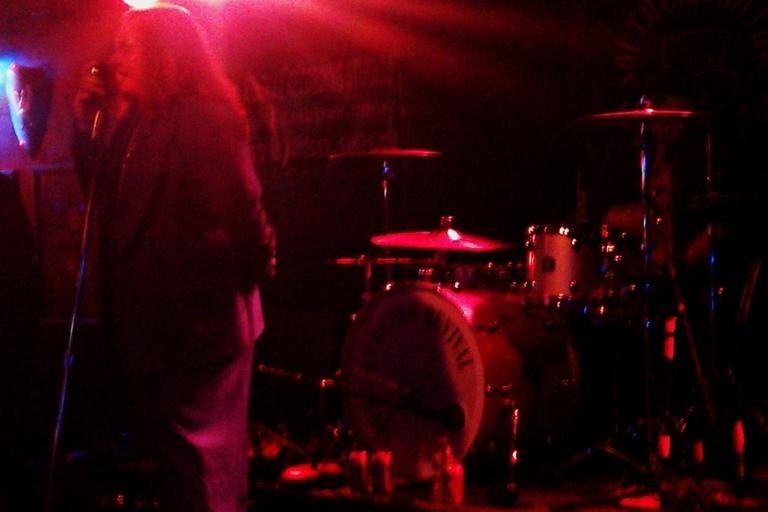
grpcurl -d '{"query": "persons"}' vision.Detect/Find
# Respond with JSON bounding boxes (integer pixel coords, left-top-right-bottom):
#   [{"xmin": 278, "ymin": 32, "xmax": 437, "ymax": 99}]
[
  {"xmin": 580, "ymin": 97, "xmax": 722, "ymax": 275},
  {"xmin": 64, "ymin": 2, "xmax": 284, "ymax": 511}
]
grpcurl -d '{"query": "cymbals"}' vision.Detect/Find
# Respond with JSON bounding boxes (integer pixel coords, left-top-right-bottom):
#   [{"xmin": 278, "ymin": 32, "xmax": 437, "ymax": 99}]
[
  {"xmin": 328, "ymin": 147, "xmax": 441, "ymax": 158},
  {"xmin": 591, "ymin": 108, "xmax": 694, "ymax": 119},
  {"xmin": 370, "ymin": 230, "xmax": 511, "ymax": 252}
]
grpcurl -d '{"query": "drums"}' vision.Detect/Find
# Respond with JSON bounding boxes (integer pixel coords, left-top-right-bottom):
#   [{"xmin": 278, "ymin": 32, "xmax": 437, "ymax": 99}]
[
  {"xmin": 590, "ymin": 223, "xmax": 659, "ymax": 315},
  {"xmin": 345, "ymin": 281, "xmax": 579, "ymax": 479},
  {"xmin": 526, "ymin": 222, "xmax": 600, "ymax": 314}
]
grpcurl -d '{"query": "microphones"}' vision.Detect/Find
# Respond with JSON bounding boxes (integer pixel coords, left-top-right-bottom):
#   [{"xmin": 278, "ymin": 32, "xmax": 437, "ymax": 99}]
[{"xmin": 91, "ymin": 62, "xmax": 114, "ymax": 112}]
